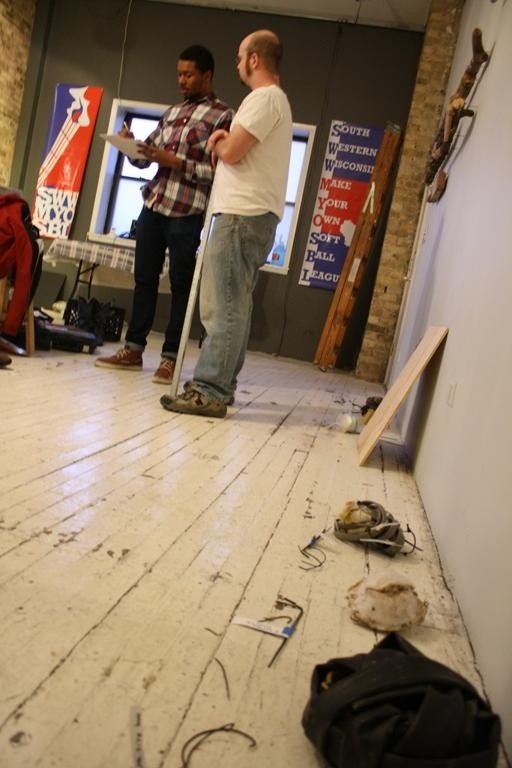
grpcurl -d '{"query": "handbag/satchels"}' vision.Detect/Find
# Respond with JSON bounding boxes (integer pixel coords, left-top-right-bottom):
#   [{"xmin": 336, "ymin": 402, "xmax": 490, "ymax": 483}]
[{"xmin": 301, "ymin": 632, "xmax": 501, "ymax": 768}]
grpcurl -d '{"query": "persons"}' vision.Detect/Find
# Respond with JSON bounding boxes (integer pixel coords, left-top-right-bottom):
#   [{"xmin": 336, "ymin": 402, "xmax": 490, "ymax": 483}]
[
  {"xmin": 95, "ymin": 44, "xmax": 234, "ymax": 385},
  {"xmin": 160, "ymin": 28, "xmax": 293, "ymax": 418}
]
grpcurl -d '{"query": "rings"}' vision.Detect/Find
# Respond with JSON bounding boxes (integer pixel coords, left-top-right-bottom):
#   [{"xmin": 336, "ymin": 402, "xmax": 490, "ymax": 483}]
[{"xmin": 152, "ymin": 152, "xmax": 156, "ymax": 157}]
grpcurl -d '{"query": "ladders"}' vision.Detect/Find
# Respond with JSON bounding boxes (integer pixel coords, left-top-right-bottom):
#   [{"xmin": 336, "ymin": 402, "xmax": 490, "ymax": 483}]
[{"xmin": 313, "ymin": 120, "xmax": 403, "ymax": 372}]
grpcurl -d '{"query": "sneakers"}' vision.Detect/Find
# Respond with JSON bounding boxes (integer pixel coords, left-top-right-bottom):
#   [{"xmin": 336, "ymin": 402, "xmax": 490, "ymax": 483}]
[
  {"xmin": 152, "ymin": 358, "xmax": 175, "ymax": 384},
  {"xmin": 161, "ymin": 381, "xmax": 235, "ymax": 418},
  {"xmin": 95, "ymin": 349, "xmax": 142, "ymax": 370}
]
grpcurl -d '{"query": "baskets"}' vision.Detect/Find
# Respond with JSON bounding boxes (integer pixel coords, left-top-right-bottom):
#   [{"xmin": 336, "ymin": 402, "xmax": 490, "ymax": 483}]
[{"xmin": 64, "ymin": 298, "xmax": 125, "ymax": 342}]
